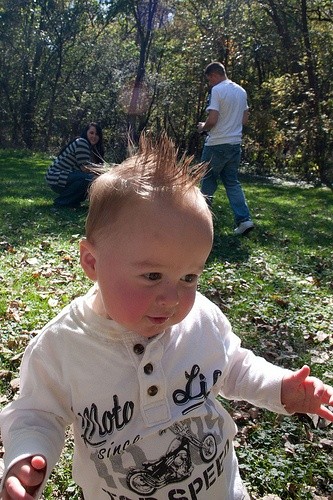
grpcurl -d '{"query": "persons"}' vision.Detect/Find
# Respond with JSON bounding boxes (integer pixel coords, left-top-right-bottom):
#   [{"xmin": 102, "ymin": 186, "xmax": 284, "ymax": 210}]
[
  {"xmin": 45, "ymin": 122, "xmax": 104, "ymax": 213},
  {"xmin": 0, "ymin": 129, "xmax": 333, "ymax": 500},
  {"xmin": 197, "ymin": 62, "xmax": 255, "ymax": 236}
]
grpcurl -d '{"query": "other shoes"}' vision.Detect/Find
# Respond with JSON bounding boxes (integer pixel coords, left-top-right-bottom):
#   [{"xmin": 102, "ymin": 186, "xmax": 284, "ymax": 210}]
[{"xmin": 236, "ymin": 221, "xmax": 253, "ymax": 234}]
